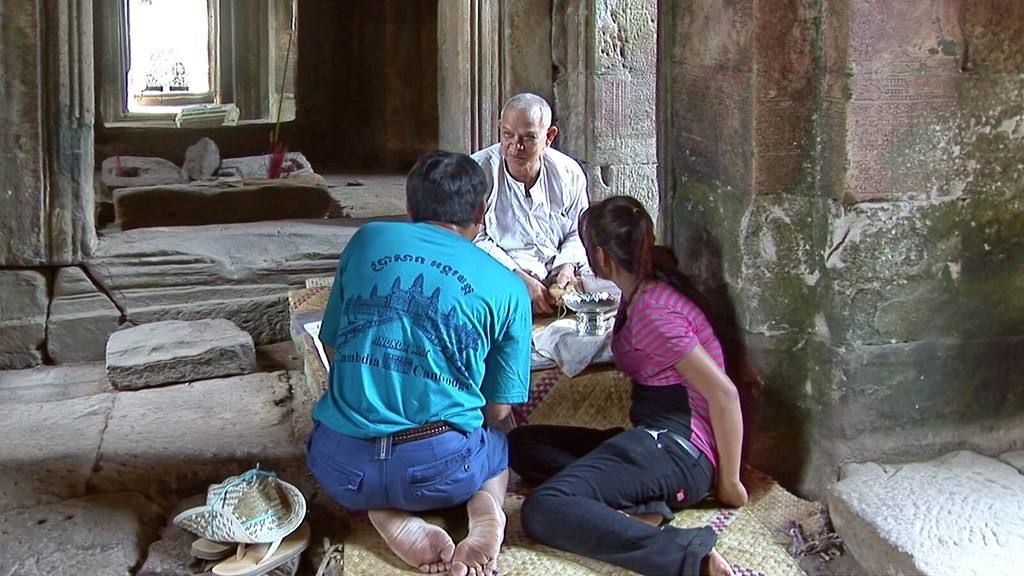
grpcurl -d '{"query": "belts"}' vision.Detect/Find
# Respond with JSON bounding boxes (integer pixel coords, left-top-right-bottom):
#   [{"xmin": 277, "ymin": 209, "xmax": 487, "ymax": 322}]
[
  {"xmin": 661, "ymin": 428, "xmax": 714, "ymax": 492},
  {"xmin": 367, "ymin": 420, "xmax": 454, "ymax": 445}
]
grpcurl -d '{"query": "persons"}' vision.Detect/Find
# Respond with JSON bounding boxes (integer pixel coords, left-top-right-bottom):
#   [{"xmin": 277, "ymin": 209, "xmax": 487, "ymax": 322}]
[
  {"xmin": 505, "ymin": 196, "xmax": 754, "ymax": 576},
  {"xmin": 304, "ymin": 149, "xmax": 532, "ymax": 576},
  {"xmin": 465, "ymin": 93, "xmax": 591, "ymax": 316}
]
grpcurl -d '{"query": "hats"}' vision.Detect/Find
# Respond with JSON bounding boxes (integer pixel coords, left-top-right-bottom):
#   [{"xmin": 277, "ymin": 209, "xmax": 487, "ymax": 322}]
[{"xmin": 173, "ymin": 468, "xmax": 306, "ymax": 543}]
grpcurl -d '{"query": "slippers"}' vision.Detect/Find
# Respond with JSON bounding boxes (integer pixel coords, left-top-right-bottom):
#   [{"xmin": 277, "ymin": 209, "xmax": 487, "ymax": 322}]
[
  {"xmin": 191, "ymin": 537, "xmax": 237, "ymax": 560},
  {"xmin": 211, "ymin": 520, "xmax": 310, "ymax": 576}
]
